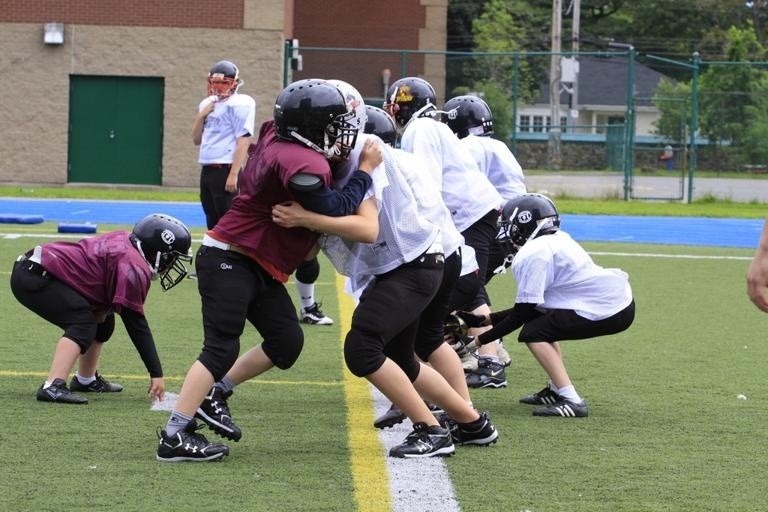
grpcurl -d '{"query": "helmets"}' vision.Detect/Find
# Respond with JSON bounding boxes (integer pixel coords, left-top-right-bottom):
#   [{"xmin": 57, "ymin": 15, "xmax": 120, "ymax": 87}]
[
  {"xmin": 495, "ymin": 194, "xmax": 560, "ymax": 246},
  {"xmin": 275, "ymin": 76, "xmax": 495, "ymax": 160},
  {"xmin": 130, "ymin": 212, "xmax": 193, "ymax": 290},
  {"xmin": 208, "ymin": 60, "xmax": 239, "ymax": 97}
]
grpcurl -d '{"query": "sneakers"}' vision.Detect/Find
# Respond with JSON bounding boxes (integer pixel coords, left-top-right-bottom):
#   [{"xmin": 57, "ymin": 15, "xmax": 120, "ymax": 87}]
[
  {"xmin": 373, "ymin": 400, "xmax": 499, "ymax": 458},
  {"xmin": 195, "ymin": 386, "xmax": 242, "ymax": 442},
  {"xmin": 156, "ymin": 417, "xmax": 228, "ymax": 462},
  {"xmin": 520, "ymin": 387, "xmax": 588, "ymax": 417},
  {"xmin": 37, "ymin": 378, "xmax": 88, "ymax": 403},
  {"xmin": 69, "ymin": 370, "xmax": 123, "ymax": 392},
  {"xmin": 463, "ymin": 338, "xmax": 512, "ymax": 388},
  {"xmin": 300, "ymin": 302, "xmax": 333, "ymax": 324}
]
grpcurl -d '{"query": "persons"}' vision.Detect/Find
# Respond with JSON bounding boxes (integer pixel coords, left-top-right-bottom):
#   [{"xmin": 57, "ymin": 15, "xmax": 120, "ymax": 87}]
[
  {"xmin": 385, "ymin": 76, "xmax": 508, "ymax": 388},
  {"xmin": 272, "ymin": 80, "xmax": 499, "ymax": 457},
  {"xmin": 746, "ymin": 216, "xmax": 768, "ymax": 313},
  {"xmin": 441, "ymin": 95, "xmax": 529, "ymax": 373},
  {"xmin": 188, "ymin": 59, "xmax": 256, "ymax": 280},
  {"xmin": 660, "ymin": 145, "xmax": 674, "ymax": 170},
  {"xmin": 11, "ymin": 214, "xmax": 191, "ymax": 404},
  {"xmin": 444, "ymin": 193, "xmax": 634, "ymax": 418},
  {"xmin": 157, "ymin": 76, "xmax": 384, "ymax": 461}
]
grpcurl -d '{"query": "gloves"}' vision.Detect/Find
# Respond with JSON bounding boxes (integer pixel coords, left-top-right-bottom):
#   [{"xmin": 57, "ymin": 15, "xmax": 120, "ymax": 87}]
[{"xmin": 442, "ymin": 309, "xmax": 486, "ymax": 358}]
